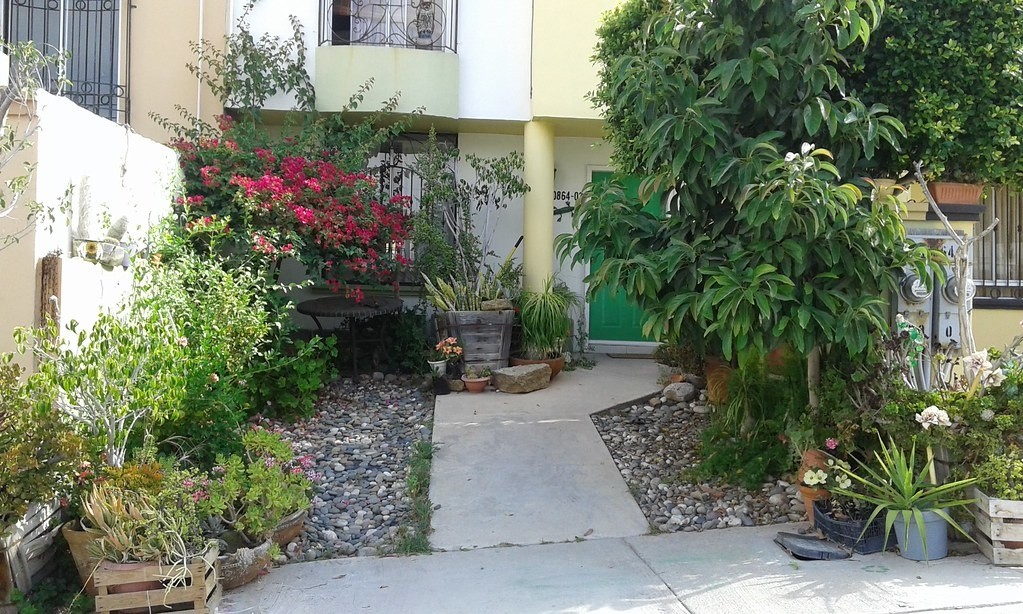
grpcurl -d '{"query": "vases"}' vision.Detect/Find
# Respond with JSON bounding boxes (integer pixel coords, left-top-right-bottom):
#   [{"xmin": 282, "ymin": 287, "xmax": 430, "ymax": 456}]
[
  {"xmin": 927, "ymin": 180, "xmax": 987, "ymax": 221},
  {"xmin": 870, "ymin": 199, "xmax": 930, "ymax": 221},
  {"xmin": 810, "ymin": 496, "xmax": 900, "ymax": 556},
  {"xmin": 60, "ymin": 517, "xmax": 115, "ymax": 596},
  {"xmin": 700, "ymin": 354, "xmax": 737, "ymax": 405},
  {"xmin": 442, "ymin": 374, "xmax": 466, "ymax": 391},
  {"xmin": 233, "ymin": 505, "xmax": 308, "ymax": 550}
]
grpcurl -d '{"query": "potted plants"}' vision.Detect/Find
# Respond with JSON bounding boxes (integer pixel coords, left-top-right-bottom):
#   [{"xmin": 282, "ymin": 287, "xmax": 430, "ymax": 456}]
[
  {"xmin": 424, "ymin": 337, "xmax": 449, "ymax": 377},
  {"xmin": 829, "ymin": 433, "xmax": 987, "ymax": 567},
  {"xmin": 963, "ymin": 414, "xmax": 1023, "ymax": 567},
  {"xmin": 506, "ymin": 272, "xmax": 582, "ymax": 380},
  {"xmin": 417, "ymin": 266, "xmax": 516, "ymax": 380},
  {"xmin": 80, "ymin": 483, "xmax": 171, "ymax": 613},
  {"xmin": 460, "ymin": 365, "xmax": 494, "ymax": 393}
]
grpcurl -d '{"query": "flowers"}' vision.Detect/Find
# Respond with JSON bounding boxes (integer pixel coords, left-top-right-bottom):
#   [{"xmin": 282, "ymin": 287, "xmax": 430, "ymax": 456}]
[
  {"xmin": 183, "ymin": 423, "xmax": 322, "ymax": 536},
  {"xmin": 802, "ymin": 435, "xmax": 864, "ymax": 516},
  {"xmin": 59, "ymin": 454, "xmax": 117, "ymax": 528},
  {"xmin": 176, "ymin": 113, "xmax": 417, "ymax": 303},
  {"xmin": 435, "ymin": 336, "xmax": 464, "ymax": 378}
]
